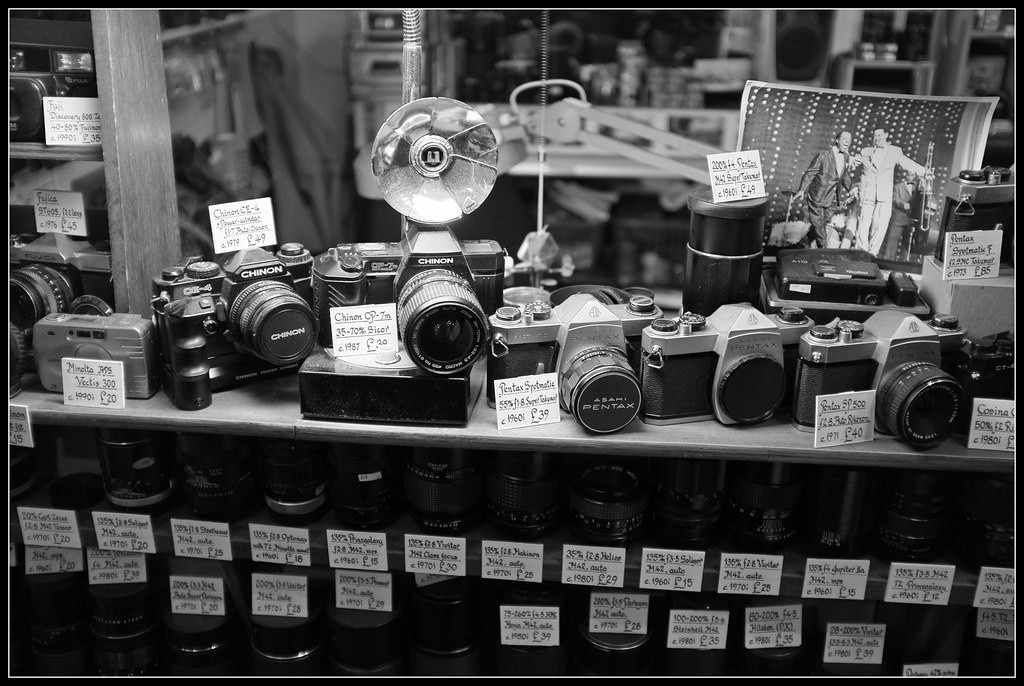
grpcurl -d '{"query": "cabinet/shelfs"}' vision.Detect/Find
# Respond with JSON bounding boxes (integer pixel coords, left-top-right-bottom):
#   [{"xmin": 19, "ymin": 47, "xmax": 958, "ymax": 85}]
[{"xmin": 10, "ymin": 11, "xmax": 1013, "ymax": 677}]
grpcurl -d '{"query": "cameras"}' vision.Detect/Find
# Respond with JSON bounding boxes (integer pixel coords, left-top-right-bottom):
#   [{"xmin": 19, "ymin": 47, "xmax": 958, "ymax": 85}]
[
  {"xmin": 9, "ymin": 46, "xmax": 97, "ymax": 143},
  {"xmin": 791, "ymin": 310, "xmax": 968, "ymax": 450},
  {"xmin": 933, "ymin": 165, "xmax": 1016, "ymax": 274},
  {"xmin": 152, "ymin": 243, "xmax": 319, "ymax": 411},
  {"xmin": 311, "ymin": 224, "xmax": 507, "ymax": 374},
  {"xmin": 641, "ymin": 302, "xmax": 816, "ymax": 426},
  {"xmin": 958, "ymin": 329, "xmax": 1015, "ymax": 433},
  {"xmin": 32, "ymin": 312, "xmax": 161, "ymax": 399},
  {"xmin": 8, "ymin": 231, "xmax": 119, "ymax": 339},
  {"xmin": 488, "ymin": 293, "xmax": 664, "ymax": 434}
]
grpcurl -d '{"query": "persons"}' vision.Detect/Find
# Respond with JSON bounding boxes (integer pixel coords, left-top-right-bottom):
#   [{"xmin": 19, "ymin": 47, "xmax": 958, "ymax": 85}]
[
  {"xmin": 845, "ymin": 127, "xmax": 935, "ymax": 261},
  {"xmin": 791, "ymin": 131, "xmax": 860, "ymax": 249}
]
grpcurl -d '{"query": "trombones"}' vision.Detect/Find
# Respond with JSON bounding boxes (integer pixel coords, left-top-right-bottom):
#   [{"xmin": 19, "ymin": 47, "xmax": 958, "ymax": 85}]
[{"xmin": 919, "ymin": 141, "xmax": 937, "ymax": 232}]
